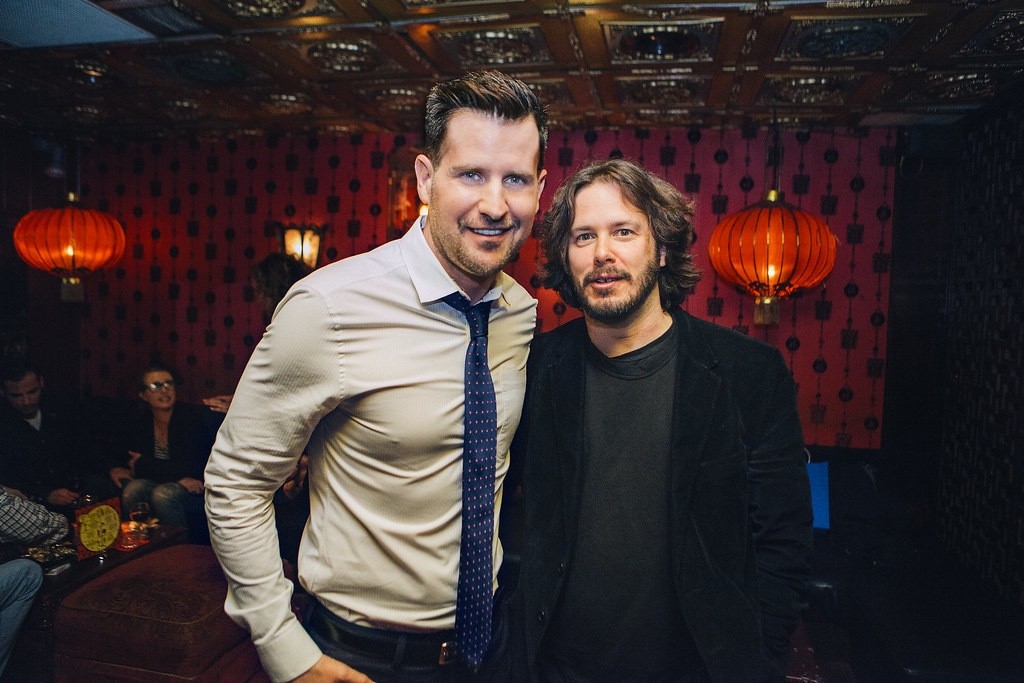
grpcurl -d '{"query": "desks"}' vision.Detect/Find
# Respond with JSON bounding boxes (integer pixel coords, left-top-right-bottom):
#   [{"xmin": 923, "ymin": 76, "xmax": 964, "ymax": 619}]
[{"xmin": 6, "ymin": 514, "xmax": 184, "ymax": 625}]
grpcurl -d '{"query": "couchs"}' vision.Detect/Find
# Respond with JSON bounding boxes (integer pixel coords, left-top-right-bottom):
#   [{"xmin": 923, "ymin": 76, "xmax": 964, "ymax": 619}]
[{"xmin": 51, "ymin": 397, "xmax": 311, "ymax": 567}]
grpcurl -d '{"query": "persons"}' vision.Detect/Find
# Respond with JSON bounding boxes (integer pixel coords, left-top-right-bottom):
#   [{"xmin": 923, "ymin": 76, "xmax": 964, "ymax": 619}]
[
  {"xmin": 0, "ymin": 483, "xmax": 71, "ymax": 682},
  {"xmin": 285, "ymin": 158, "xmax": 817, "ymax": 683},
  {"xmin": 201, "ymin": 72, "xmax": 551, "ymax": 682},
  {"xmin": 0, "ymin": 359, "xmax": 134, "ymax": 544},
  {"xmin": 117, "ymin": 362, "xmax": 210, "ymax": 545},
  {"xmin": 203, "ymin": 252, "xmax": 308, "ymax": 412}
]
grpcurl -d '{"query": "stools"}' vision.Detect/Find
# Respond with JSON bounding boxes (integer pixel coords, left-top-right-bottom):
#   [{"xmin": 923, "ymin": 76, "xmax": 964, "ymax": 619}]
[{"xmin": 56, "ymin": 540, "xmax": 299, "ymax": 683}]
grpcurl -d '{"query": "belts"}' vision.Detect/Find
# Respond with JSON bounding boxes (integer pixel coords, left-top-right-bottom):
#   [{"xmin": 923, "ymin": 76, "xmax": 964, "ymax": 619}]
[{"xmin": 301, "ymin": 598, "xmax": 455, "ymax": 665}]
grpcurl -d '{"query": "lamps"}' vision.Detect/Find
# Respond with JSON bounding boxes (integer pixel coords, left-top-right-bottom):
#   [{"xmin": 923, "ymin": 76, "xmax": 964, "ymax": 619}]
[{"xmin": 280, "ymin": 224, "xmax": 326, "ymax": 279}]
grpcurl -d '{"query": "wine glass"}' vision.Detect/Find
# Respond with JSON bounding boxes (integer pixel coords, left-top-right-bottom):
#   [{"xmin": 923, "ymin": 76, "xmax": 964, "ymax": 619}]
[{"xmin": 129, "ymin": 503, "xmax": 151, "ymax": 533}]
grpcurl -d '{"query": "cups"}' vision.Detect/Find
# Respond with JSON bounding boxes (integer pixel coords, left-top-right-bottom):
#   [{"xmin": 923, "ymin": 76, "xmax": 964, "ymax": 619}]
[{"xmin": 119, "ymin": 521, "xmax": 140, "ymax": 549}]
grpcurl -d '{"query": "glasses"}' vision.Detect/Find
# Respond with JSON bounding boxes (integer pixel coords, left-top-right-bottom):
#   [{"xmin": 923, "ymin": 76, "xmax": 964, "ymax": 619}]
[{"xmin": 143, "ymin": 381, "xmax": 175, "ymax": 391}]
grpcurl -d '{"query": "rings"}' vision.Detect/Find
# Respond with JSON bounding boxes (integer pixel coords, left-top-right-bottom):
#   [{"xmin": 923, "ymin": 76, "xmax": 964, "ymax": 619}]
[{"xmin": 220, "ymin": 399, "xmax": 225, "ymax": 403}]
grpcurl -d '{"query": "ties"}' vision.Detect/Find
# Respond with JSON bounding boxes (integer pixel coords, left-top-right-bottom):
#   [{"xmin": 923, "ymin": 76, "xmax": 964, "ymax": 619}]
[{"xmin": 441, "ymin": 290, "xmax": 498, "ymax": 677}]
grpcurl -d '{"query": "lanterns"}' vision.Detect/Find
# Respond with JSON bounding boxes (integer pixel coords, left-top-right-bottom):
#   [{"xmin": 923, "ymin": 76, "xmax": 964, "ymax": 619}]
[
  {"xmin": 12, "ymin": 197, "xmax": 126, "ymax": 286},
  {"xmin": 708, "ymin": 193, "xmax": 838, "ymax": 325}
]
